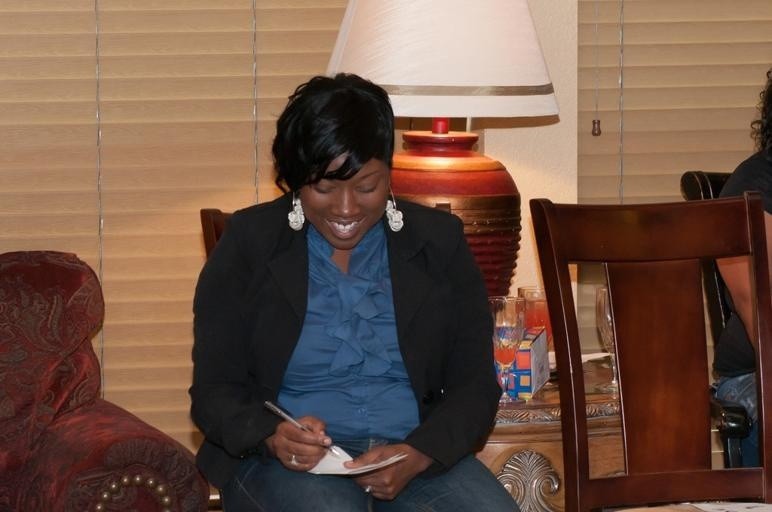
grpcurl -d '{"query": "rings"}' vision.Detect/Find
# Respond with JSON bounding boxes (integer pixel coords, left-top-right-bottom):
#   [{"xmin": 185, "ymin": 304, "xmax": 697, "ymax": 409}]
[
  {"xmin": 365, "ymin": 484, "xmax": 373, "ymax": 494},
  {"xmin": 290, "ymin": 453, "xmax": 300, "ymax": 466}
]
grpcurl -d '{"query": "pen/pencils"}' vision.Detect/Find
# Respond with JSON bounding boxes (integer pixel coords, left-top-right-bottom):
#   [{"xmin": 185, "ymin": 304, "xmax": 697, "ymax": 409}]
[{"xmin": 264, "ymin": 401, "xmax": 342, "ymax": 458}]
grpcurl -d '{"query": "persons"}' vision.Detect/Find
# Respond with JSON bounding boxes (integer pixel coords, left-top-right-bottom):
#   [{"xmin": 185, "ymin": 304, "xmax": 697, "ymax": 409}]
[
  {"xmin": 705, "ymin": 69, "xmax": 772, "ymax": 488},
  {"xmin": 188, "ymin": 70, "xmax": 523, "ymax": 511}
]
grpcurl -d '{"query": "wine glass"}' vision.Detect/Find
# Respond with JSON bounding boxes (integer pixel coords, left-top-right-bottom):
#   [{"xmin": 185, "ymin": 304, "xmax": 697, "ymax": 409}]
[
  {"xmin": 593, "ymin": 286, "xmax": 619, "ymax": 391},
  {"xmin": 489, "ymin": 295, "xmax": 528, "ymax": 404}
]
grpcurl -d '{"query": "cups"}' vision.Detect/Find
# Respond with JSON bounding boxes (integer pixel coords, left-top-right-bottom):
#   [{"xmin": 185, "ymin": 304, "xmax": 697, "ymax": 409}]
[{"xmin": 514, "ymin": 287, "xmax": 557, "ymax": 373}]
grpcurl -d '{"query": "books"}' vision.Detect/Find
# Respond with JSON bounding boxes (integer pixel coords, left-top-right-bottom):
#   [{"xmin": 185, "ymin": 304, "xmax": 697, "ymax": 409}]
[{"xmin": 307, "ymin": 443, "xmax": 410, "ymax": 477}]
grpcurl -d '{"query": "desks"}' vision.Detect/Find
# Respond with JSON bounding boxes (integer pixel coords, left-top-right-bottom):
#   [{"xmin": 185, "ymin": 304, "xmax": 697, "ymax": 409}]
[{"xmin": 474, "ymin": 348, "xmax": 726, "ymax": 512}]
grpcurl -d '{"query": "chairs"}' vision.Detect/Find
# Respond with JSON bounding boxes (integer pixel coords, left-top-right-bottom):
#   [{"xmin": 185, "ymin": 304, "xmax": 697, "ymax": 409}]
[
  {"xmin": 201, "ymin": 208, "xmax": 237, "ymax": 261},
  {"xmin": 0, "ymin": 252, "xmax": 211, "ymax": 512},
  {"xmin": 529, "ymin": 191, "xmax": 772, "ymax": 511},
  {"xmin": 680, "ymin": 171, "xmax": 753, "ymax": 470}
]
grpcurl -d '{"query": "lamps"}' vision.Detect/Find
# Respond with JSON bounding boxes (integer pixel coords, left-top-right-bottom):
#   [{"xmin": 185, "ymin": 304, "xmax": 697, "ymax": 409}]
[{"xmin": 320, "ymin": 0, "xmax": 560, "ymax": 331}]
